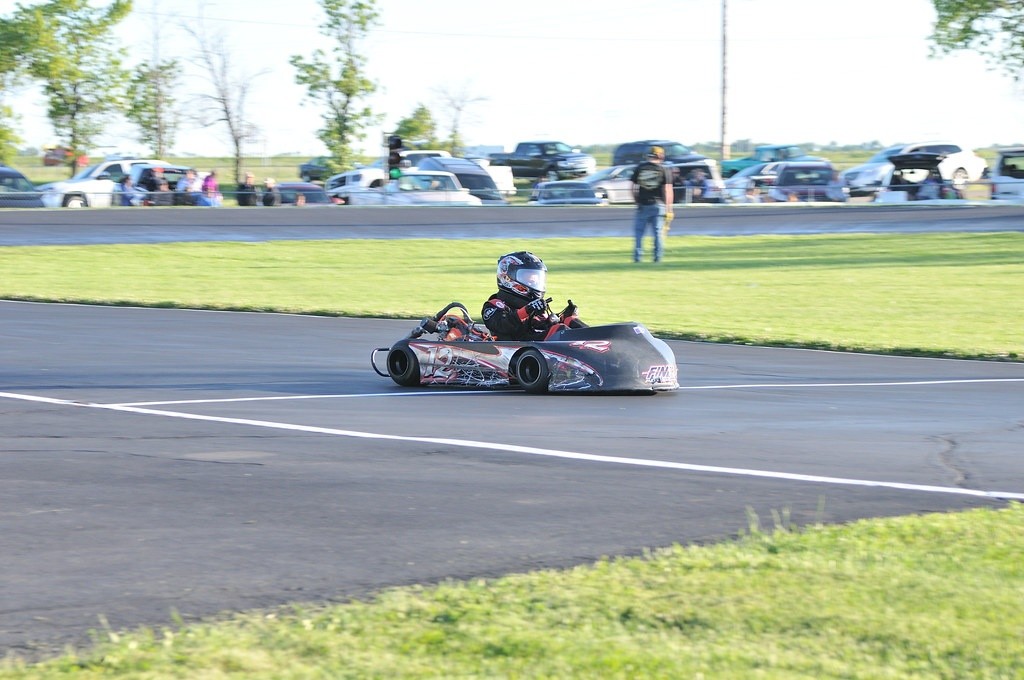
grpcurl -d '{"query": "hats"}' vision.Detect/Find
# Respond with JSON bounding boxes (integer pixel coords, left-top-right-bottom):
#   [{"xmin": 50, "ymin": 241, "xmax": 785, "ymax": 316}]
[{"xmin": 645, "ymin": 145, "xmax": 665, "ymax": 157}]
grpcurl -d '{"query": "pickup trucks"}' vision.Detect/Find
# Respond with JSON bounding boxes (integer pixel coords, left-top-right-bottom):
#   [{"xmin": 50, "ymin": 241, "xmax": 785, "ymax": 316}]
[
  {"xmin": 720, "ymin": 144, "xmax": 830, "ymax": 178},
  {"xmin": 489, "ymin": 140, "xmax": 598, "ymax": 181}
]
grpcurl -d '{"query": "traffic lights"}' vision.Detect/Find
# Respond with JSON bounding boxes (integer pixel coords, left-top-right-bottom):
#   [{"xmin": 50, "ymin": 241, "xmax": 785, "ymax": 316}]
[{"xmin": 388, "ymin": 135, "xmax": 403, "ymax": 179}]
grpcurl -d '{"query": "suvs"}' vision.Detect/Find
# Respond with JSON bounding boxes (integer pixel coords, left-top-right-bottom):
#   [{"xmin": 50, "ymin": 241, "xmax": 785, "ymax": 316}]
[
  {"xmin": 670, "ymin": 158, "xmax": 725, "ymax": 203},
  {"xmin": 874, "ymin": 152, "xmax": 968, "ymax": 200},
  {"xmin": 0, "ymin": 162, "xmax": 46, "ymax": 209},
  {"xmin": 34, "ymin": 158, "xmax": 224, "ymax": 210},
  {"xmin": 613, "ymin": 139, "xmax": 721, "ymax": 178},
  {"xmin": 837, "ymin": 140, "xmax": 964, "ymax": 196},
  {"xmin": 727, "ymin": 158, "xmax": 845, "ymax": 204}
]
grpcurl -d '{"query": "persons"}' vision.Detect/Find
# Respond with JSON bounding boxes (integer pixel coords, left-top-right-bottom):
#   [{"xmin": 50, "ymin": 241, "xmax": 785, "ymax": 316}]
[
  {"xmin": 120, "ymin": 174, "xmax": 148, "ymax": 206},
  {"xmin": 295, "ymin": 194, "xmax": 305, "ymax": 207},
  {"xmin": 672, "ymin": 168, "xmax": 707, "ymax": 203},
  {"xmin": 237, "ymin": 172, "xmax": 256, "ymax": 206},
  {"xmin": 144, "ymin": 167, "xmax": 174, "ymax": 205},
  {"xmin": 916, "ymin": 172, "xmax": 940, "ymax": 199},
  {"xmin": 175, "ymin": 170, "xmax": 222, "ymax": 207},
  {"xmin": 482, "ymin": 252, "xmax": 589, "ymax": 341},
  {"xmin": 631, "ymin": 146, "xmax": 674, "ymax": 264},
  {"xmin": 256, "ymin": 178, "xmax": 281, "ymax": 205}
]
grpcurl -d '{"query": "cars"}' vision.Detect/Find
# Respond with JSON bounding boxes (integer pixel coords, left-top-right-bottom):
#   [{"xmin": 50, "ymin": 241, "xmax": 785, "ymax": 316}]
[
  {"xmin": 265, "ymin": 181, "xmax": 343, "ymax": 206},
  {"xmin": 990, "ymin": 146, "xmax": 1024, "ymax": 203},
  {"xmin": 530, "ymin": 178, "xmax": 606, "ymax": 206},
  {"xmin": 299, "ymin": 155, "xmax": 364, "ymax": 183},
  {"xmin": 578, "ymin": 160, "xmax": 681, "ymax": 204},
  {"xmin": 325, "ymin": 150, "xmax": 508, "ymax": 206}
]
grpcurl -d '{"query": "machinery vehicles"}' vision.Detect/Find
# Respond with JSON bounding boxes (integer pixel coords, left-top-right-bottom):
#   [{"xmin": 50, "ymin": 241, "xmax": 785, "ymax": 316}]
[{"xmin": 43, "ymin": 143, "xmax": 89, "ymax": 167}]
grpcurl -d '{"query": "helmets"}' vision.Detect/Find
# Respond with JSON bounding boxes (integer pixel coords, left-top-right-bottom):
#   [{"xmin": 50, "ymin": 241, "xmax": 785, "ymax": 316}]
[{"xmin": 496, "ymin": 251, "xmax": 547, "ymax": 301}]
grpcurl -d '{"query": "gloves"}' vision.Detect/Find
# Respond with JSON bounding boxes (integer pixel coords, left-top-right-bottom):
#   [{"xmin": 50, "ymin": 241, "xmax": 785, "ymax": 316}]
[{"xmin": 525, "ymin": 299, "xmax": 546, "ymax": 316}]
